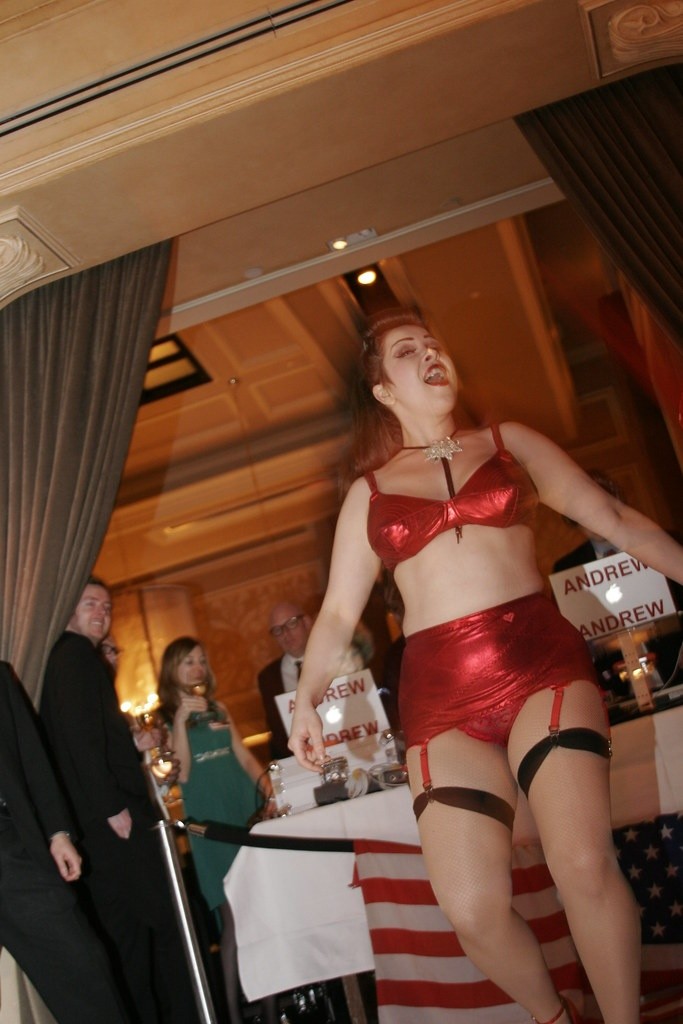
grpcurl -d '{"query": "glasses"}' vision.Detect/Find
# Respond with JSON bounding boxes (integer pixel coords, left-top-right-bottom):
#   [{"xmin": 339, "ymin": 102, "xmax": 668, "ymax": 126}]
[
  {"xmin": 270, "ymin": 615, "xmax": 303, "ymax": 637},
  {"xmin": 100, "ymin": 644, "xmax": 120, "ymax": 655}
]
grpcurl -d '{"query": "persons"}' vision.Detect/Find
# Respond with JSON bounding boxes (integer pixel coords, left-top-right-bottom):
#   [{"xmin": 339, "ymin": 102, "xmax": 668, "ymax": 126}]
[
  {"xmin": 0, "ymin": 575, "xmax": 389, "ymax": 1024},
  {"xmin": 286, "ymin": 304, "xmax": 683, "ymax": 1024}
]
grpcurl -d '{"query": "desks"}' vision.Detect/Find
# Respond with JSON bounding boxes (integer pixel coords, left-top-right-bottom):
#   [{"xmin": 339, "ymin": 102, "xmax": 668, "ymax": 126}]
[{"xmin": 224, "ymin": 683, "xmax": 683, "ymax": 1024}]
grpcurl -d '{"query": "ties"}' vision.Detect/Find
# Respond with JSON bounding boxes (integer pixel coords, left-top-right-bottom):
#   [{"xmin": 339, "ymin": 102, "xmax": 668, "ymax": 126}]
[{"xmin": 295, "ymin": 662, "xmax": 302, "ymax": 680}]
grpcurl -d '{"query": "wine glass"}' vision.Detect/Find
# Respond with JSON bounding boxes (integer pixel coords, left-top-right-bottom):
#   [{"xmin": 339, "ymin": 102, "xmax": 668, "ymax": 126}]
[
  {"xmin": 133, "ymin": 706, "xmax": 166, "ymax": 764},
  {"xmin": 186, "ymin": 667, "xmax": 216, "ymax": 720}
]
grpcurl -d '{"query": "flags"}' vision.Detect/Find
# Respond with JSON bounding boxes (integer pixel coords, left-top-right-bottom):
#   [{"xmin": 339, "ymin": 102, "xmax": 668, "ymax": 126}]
[{"xmin": 351, "ymin": 809, "xmax": 683, "ymax": 1024}]
